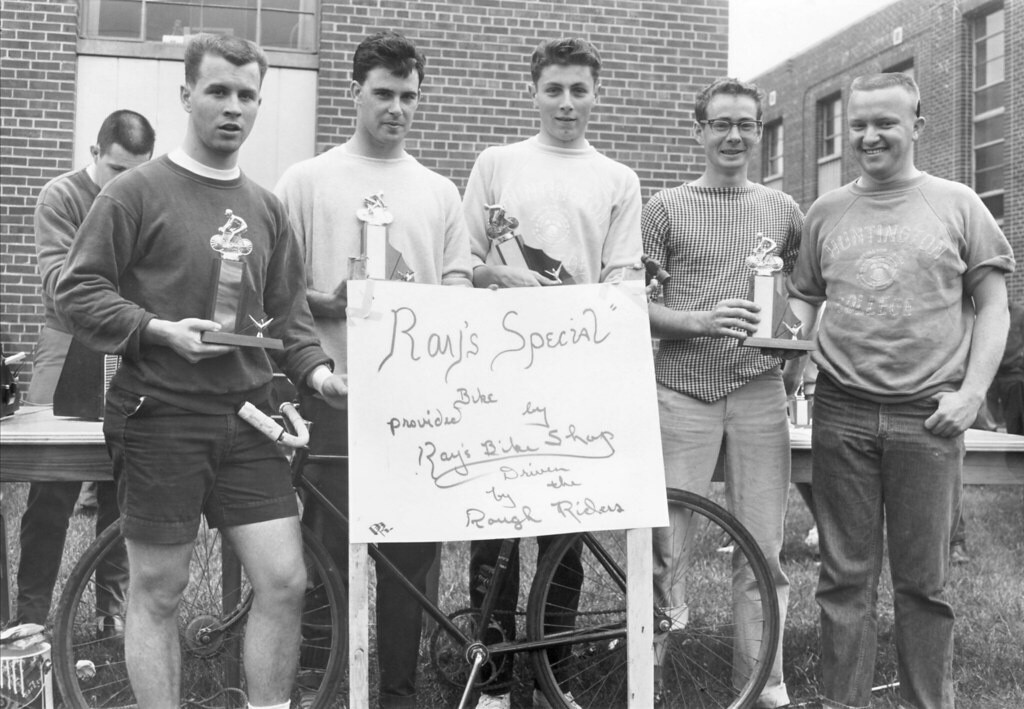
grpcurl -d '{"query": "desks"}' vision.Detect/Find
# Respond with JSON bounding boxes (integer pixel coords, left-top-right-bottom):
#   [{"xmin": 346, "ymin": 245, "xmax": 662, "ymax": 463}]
[{"xmin": 0, "ymin": 403, "xmax": 1024, "ymax": 709}]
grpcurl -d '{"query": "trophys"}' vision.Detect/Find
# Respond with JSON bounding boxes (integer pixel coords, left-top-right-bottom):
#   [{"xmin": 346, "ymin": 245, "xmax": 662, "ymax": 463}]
[
  {"xmin": 483, "ymin": 203, "xmax": 534, "ymax": 270},
  {"xmin": 357, "ymin": 192, "xmax": 393, "ymax": 280},
  {"xmin": 737, "ymin": 233, "xmax": 817, "ymax": 351},
  {"xmin": 202, "ymin": 208, "xmax": 283, "ymax": 349}
]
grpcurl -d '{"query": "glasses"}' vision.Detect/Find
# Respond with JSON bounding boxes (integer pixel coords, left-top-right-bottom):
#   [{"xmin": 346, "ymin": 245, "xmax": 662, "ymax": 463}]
[{"xmin": 700, "ymin": 119, "xmax": 763, "ymax": 134}]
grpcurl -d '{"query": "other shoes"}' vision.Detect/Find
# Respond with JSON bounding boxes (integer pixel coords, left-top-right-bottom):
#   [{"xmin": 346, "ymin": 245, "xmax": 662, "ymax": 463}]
[{"xmin": 94, "ymin": 618, "xmax": 126, "ymax": 649}]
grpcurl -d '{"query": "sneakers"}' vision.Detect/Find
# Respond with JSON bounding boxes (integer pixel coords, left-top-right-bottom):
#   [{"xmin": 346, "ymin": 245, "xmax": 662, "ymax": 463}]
[
  {"xmin": 532, "ymin": 690, "xmax": 581, "ymax": 709},
  {"xmin": 477, "ymin": 693, "xmax": 508, "ymax": 709}
]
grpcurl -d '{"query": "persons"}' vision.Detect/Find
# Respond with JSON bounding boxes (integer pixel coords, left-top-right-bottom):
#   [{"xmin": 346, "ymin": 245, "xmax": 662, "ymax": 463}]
[
  {"xmin": 271, "ymin": 31, "xmax": 499, "ymax": 709},
  {"xmin": 643, "ymin": 77, "xmax": 803, "ymax": 709},
  {"xmin": 52, "ymin": 31, "xmax": 350, "ymax": 709},
  {"xmin": 782, "ymin": 73, "xmax": 1018, "ymax": 708},
  {"xmin": 789, "ymin": 301, "xmax": 1023, "ymax": 562},
  {"xmin": 462, "ymin": 38, "xmax": 642, "ymax": 709},
  {"xmin": 16, "ymin": 111, "xmax": 157, "ymax": 652}
]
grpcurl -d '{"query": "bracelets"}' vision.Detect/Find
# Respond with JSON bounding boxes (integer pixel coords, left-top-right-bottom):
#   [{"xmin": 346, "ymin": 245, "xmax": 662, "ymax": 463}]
[{"xmin": 306, "ymin": 365, "xmax": 334, "ymax": 397}]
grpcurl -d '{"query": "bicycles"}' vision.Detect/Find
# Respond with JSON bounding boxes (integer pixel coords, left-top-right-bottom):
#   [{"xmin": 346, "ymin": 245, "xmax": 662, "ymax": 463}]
[{"xmin": 50, "ymin": 400, "xmax": 780, "ymax": 709}]
